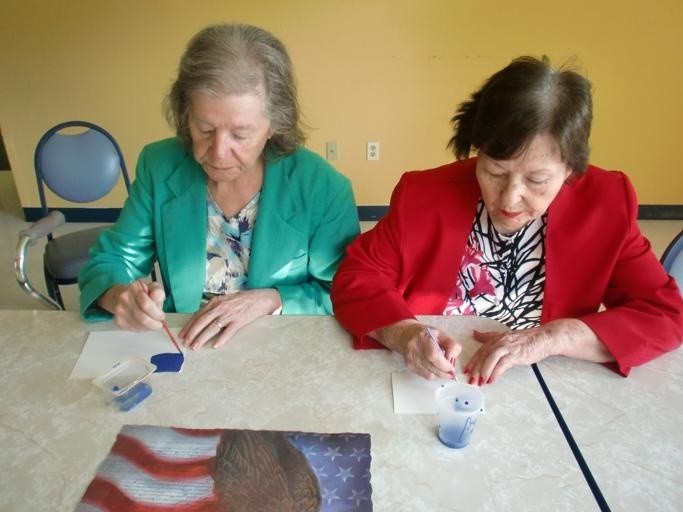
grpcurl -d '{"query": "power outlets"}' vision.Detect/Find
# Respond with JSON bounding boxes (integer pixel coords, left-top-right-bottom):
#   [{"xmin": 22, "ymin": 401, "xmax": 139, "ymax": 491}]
[{"xmin": 367, "ymin": 142, "xmax": 379, "ymax": 160}]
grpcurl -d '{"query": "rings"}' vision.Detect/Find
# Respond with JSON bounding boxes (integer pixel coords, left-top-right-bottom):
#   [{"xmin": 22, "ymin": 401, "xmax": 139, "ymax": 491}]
[{"xmin": 215, "ymin": 321, "xmax": 225, "ymax": 331}]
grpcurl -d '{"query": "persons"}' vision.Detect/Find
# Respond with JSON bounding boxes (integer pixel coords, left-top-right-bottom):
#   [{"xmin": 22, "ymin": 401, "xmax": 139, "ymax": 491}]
[
  {"xmin": 331, "ymin": 51, "xmax": 683, "ymax": 388},
  {"xmin": 76, "ymin": 22, "xmax": 360, "ymax": 350}
]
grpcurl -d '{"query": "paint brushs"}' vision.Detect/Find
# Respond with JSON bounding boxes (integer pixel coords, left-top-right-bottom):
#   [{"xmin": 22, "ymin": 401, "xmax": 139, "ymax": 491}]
[{"xmin": 134, "ymin": 278, "xmax": 185, "ymax": 354}]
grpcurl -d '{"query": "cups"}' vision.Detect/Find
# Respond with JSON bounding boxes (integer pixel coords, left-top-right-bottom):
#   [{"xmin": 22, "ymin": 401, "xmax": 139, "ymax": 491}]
[{"xmin": 434, "ymin": 382, "xmax": 485, "ymax": 448}]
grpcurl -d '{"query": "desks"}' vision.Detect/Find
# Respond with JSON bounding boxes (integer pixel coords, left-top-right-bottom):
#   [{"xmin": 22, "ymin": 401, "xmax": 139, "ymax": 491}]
[{"xmin": 0, "ymin": 310, "xmax": 683, "ymax": 512}]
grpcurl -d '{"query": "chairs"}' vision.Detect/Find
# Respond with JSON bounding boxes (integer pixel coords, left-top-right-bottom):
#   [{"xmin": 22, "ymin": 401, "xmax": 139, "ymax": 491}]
[{"xmin": 33, "ymin": 118, "xmax": 134, "ymax": 311}]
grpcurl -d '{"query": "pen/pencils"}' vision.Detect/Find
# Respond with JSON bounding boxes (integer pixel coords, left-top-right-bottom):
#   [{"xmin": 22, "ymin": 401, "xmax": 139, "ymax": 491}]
[{"xmin": 425, "ymin": 329, "xmax": 462, "ymax": 380}]
